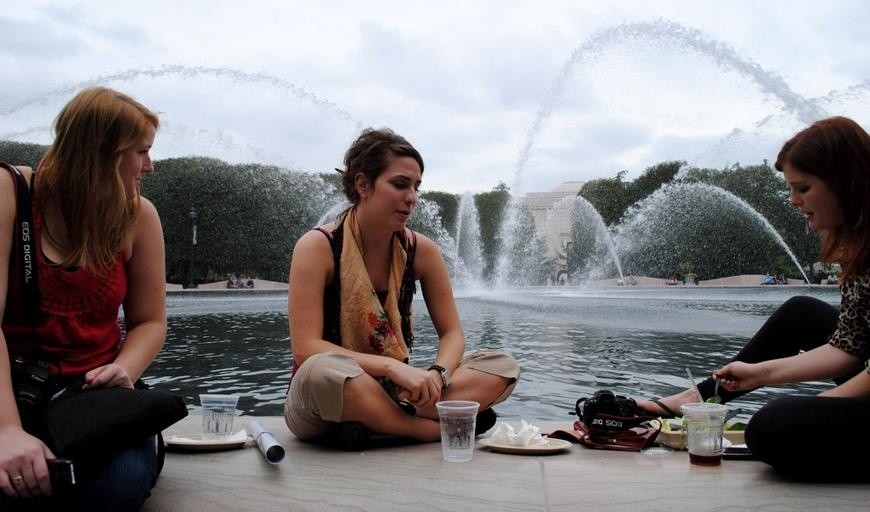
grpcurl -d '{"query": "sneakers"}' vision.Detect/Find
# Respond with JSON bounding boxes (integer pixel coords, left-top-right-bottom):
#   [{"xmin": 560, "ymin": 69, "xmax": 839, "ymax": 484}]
[
  {"xmin": 476, "ymin": 409, "xmax": 496, "ymax": 435},
  {"xmin": 332, "ymin": 421, "xmax": 387, "ymax": 452}
]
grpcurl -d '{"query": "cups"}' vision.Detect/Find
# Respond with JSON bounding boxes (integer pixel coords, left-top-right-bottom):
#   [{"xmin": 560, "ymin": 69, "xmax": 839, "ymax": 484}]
[
  {"xmin": 436, "ymin": 400, "xmax": 480, "ymax": 463},
  {"xmin": 679, "ymin": 402, "xmax": 729, "ymax": 465},
  {"xmin": 198, "ymin": 394, "xmax": 240, "ymax": 441}
]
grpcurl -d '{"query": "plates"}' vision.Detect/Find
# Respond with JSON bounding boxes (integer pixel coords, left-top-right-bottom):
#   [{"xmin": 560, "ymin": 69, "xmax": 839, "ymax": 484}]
[
  {"xmin": 479, "ymin": 438, "xmax": 573, "ymax": 453},
  {"xmin": 162, "ymin": 435, "xmax": 246, "ymax": 450}
]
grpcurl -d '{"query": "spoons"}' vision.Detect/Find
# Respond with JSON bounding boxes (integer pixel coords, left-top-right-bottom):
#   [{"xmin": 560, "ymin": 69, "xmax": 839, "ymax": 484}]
[{"xmin": 703, "ymin": 375, "xmax": 722, "ymax": 404}]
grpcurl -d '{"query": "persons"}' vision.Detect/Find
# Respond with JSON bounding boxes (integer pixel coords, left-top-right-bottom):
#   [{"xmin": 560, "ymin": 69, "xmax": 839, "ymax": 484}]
[
  {"xmin": 246, "ymin": 276, "xmax": 254, "ymax": 289},
  {"xmin": 235, "ymin": 277, "xmax": 245, "ymax": 289},
  {"xmin": 281, "ymin": 124, "xmax": 523, "ymax": 452},
  {"xmin": 763, "ymin": 271, "xmax": 785, "ymax": 285},
  {"xmin": 665, "ymin": 274, "xmax": 678, "ymax": 285},
  {"xmin": 628, "ymin": 113, "xmax": 869, "ymax": 487},
  {"xmin": 617, "ymin": 277, "xmax": 624, "ymax": 287},
  {"xmin": 226, "ymin": 276, "xmax": 235, "ymax": 289},
  {"xmin": 1, "ymin": 85, "xmax": 171, "ymax": 511}
]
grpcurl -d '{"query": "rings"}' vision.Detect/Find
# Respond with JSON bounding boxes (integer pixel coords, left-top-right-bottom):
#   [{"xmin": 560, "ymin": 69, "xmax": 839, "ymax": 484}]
[{"xmin": 12, "ymin": 475, "xmax": 23, "ymax": 484}]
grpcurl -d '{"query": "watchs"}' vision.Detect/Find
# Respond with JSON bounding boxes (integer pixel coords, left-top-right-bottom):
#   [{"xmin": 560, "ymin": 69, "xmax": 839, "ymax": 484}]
[{"xmin": 427, "ymin": 364, "xmax": 452, "ymax": 389}]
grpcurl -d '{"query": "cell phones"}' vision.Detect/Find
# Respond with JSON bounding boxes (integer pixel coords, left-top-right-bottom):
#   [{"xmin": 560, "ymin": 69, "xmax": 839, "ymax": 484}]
[{"xmin": 44, "ymin": 457, "xmax": 77, "ymax": 485}]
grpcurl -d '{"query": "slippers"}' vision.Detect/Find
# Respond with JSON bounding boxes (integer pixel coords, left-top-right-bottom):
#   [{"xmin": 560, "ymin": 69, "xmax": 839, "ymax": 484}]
[{"xmin": 637, "ymin": 398, "xmax": 683, "ymax": 419}]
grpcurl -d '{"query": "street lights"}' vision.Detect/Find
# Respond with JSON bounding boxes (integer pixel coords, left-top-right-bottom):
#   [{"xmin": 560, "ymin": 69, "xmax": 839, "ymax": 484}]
[{"xmin": 188, "ymin": 206, "xmax": 199, "ymax": 283}]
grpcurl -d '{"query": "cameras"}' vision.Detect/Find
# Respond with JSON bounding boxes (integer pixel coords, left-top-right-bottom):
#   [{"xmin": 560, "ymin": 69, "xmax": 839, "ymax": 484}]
[{"xmin": 584, "ymin": 391, "xmax": 637, "ymax": 440}]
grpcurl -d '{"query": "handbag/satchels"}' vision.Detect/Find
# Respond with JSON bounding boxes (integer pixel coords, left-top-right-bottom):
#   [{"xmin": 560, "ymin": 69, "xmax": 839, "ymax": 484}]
[{"xmin": 46, "ymin": 376, "xmax": 189, "ymax": 486}]
